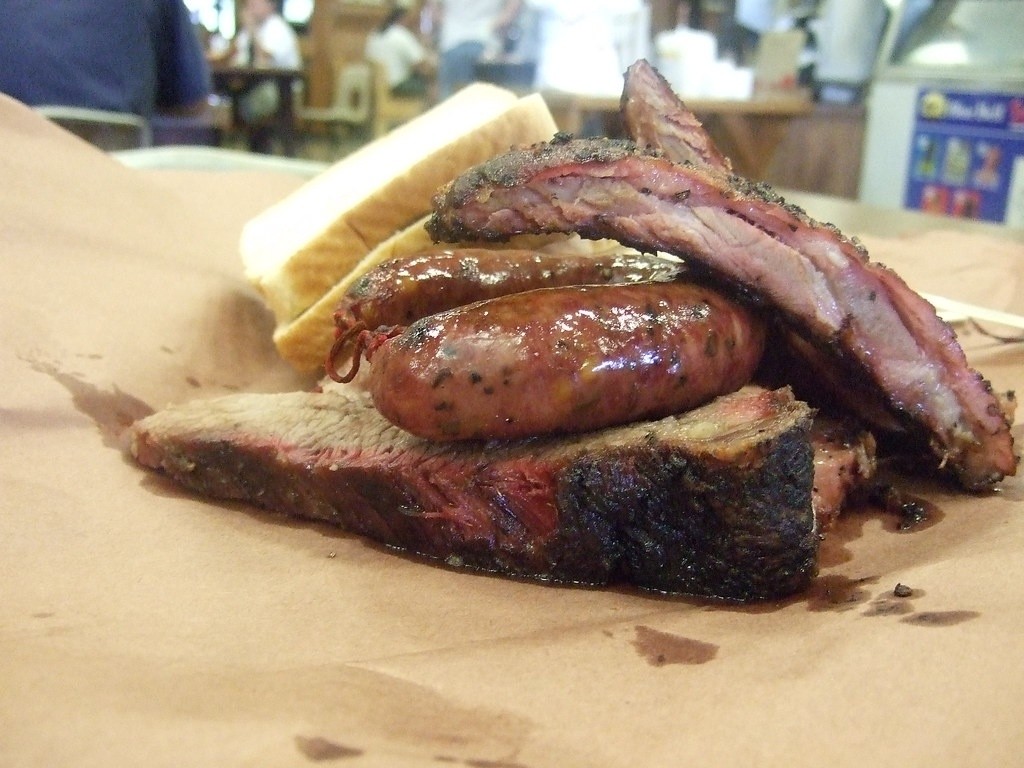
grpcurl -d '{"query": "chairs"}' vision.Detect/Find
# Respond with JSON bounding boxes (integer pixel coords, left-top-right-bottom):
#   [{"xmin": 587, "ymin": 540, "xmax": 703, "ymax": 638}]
[{"xmin": 366, "ymin": 59, "xmax": 420, "ymax": 142}]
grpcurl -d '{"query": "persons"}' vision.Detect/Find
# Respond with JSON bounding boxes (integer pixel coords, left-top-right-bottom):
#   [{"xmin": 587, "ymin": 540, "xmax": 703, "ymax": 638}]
[
  {"xmin": 362, "ymin": 3, "xmax": 430, "ymax": 97},
  {"xmin": 475, "ymin": 0, "xmax": 546, "ymax": 94},
  {"xmin": 1, "ymin": 1, "xmax": 214, "ymax": 147},
  {"xmin": 206, "ymin": 0, "xmax": 305, "ymax": 120}
]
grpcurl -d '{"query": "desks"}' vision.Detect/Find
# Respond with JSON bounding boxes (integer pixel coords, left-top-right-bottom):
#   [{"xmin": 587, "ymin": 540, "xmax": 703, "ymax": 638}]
[
  {"xmin": 223, "ymin": 65, "xmax": 303, "ymax": 157},
  {"xmin": 529, "ymin": 97, "xmax": 814, "ymax": 198}
]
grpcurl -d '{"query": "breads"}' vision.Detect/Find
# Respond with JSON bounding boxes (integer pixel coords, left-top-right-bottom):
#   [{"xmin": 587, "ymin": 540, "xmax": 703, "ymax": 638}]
[{"xmin": 234, "ymin": 82, "xmax": 579, "ymax": 375}]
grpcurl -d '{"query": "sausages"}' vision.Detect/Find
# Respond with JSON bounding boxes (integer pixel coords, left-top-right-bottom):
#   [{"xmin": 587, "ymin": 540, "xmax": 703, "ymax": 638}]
[
  {"xmin": 331, "ymin": 249, "xmax": 686, "ymax": 350},
  {"xmin": 364, "ymin": 279, "xmax": 767, "ymax": 443}
]
